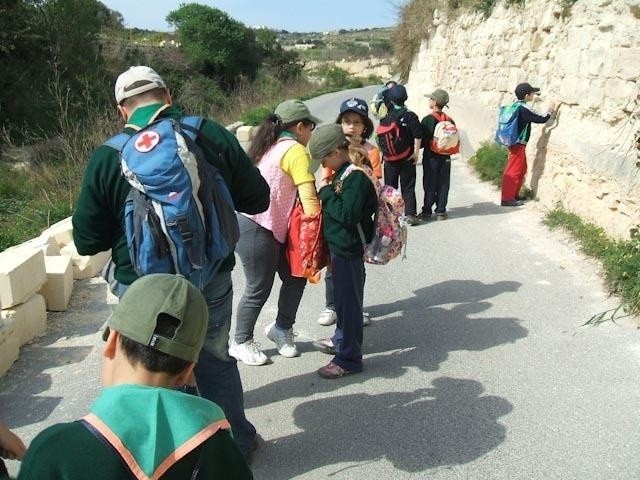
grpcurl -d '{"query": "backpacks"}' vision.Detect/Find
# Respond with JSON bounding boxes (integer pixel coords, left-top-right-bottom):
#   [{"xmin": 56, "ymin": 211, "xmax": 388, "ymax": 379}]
[
  {"xmin": 494, "ymin": 103, "xmax": 529, "ymax": 147},
  {"xmin": 431, "ymin": 110, "xmax": 460, "ymax": 156},
  {"xmin": 377, "ymin": 109, "xmax": 414, "ymax": 162},
  {"xmin": 104, "ymin": 114, "xmax": 239, "ymax": 287}
]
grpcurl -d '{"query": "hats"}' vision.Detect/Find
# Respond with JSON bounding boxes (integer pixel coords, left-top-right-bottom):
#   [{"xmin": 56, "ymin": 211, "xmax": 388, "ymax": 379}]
[
  {"xmin": 516, "ymin": 83, "xmax": 540, "ymax": 99},
  {"xmin": 101, "ymin": 272, "xmax": 210, "ymax": 364},
  {"xmin": 387, "ymin": 84, "xmax": 408, "ymax": 103},
  {"xmin": 271, "ymin": 98, "xmax": 367, "ymax": 173},
  {"xmin": 114, "ymin": 65, "xmax": 166, "ymax": 104},
  {"xmin": 424, "ymin": 89, "xmax": 449, "ymax": 106}
]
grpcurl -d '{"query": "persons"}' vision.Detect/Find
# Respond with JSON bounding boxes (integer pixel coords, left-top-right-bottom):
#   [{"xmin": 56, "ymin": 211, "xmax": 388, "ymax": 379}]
[
  {"xmin": 308, "ymin": 125, "xmax": 379, "ymax": 379},
  {"xmin": 72, "ymin": 65, "xmax": 269, "ymax": 465},
  {"xmin": 373, "ymin": 80, "xmax": 396, "ymax": 119},
  {"xmin": 380, "ymin": 84, "xmax": 422, "ymax": 225},
  {"xmin": 501, "ymin": 82, "xmax": 556, "ymax": 207},
  {"xmin": 417, "ymin": 87, "xmax": 456, "ymax": 222},
  {"xmin": 317, "ymin": 99, "xmax": 381, "ymax": 328},
  {"xmin": 1, "ymin": 421, "xmax": 30, "ymax": 480},
  {"xmin": 226, "ymin": 98, "xmax": 322, "ymax": 365},
  {"xmin": 18, "ymin": 271, "xmax": 255, "ymax": 479}
]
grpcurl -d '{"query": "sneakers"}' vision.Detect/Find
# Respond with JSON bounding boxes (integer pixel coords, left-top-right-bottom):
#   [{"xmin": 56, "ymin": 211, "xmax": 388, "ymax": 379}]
[
  {"xmin": 516, "ymin": 195, "xmax": 527, "ymax": 201},
  {"xmin": 502, "ymin": 199, "xmax": 523, "ymax": 206},
  {"xmin": 229, "ymin": 340, "xmax": 267, "ymax": 365},
  {"xmin": 265, "ymin": 322, "xmax": 298, "ymax": 357},
  {"xmin": 409, "ymin": 211, "xmax": 448, "ymax": 224},
  {"xmin": 313, "ymin": 307, "xmax": 370, "ymax": 378}
]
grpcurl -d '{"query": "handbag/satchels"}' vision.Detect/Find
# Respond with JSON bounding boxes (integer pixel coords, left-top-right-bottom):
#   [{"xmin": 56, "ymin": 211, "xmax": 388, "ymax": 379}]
[
  {"xmin": 341, "ymin": 165, "xmax": 408, "ymax": 265},
  {"xmin": 284, "ymin": 199, "xmax": 329, "ymax": 278}
]
